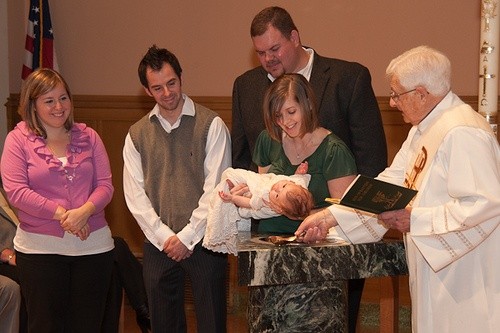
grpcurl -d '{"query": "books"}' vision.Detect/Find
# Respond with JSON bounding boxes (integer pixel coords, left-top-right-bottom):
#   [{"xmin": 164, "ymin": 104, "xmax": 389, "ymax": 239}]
[{"xmin": 325, "ymin": 174, "xmax": 418, "ymax": 215}]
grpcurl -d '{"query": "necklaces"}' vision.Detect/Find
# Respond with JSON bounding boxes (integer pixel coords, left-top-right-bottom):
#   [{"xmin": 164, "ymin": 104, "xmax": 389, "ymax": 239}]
[{"xmin": 297, "ymin": 154, "xmax": 300, "ymax": 159}]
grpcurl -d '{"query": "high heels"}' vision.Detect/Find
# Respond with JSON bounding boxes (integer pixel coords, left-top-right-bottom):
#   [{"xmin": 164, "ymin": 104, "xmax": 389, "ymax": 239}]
[{"xmin": 136, "ymin": 304, "xmax": 151, "ymax": 333}]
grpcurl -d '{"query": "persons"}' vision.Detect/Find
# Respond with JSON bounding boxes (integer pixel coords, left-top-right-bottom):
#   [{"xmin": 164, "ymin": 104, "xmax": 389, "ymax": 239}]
[
  {"xmin": 0, "ymin": 180, "xmax": 150, "ymax": 333},
  {"xmin": 231, "ymin": 6, "xmax": 388, "ymax": 333},
  {"xmin": 294, "ymin": 46, "xmax": 500, "ymax": 333},
  {"xmin": 252, "ymin": 73, "xmax": 357, "ymax": 234},
  {"xmin": 123, "ymin": 44, "xmax": 232, "ymax": 333},
  {"xmin": 0, "ymin": 68, "xmax": 119, "ymax": 333},
  {"xmin": 218, "ymin": 161, "xmax": 313, "ymax": 220}
]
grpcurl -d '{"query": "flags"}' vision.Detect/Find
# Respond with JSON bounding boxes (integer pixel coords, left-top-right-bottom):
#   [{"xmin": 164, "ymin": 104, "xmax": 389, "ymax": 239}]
[{"xmin": 22, "ymin": 0, "xmax": 60, "ymax": 82}]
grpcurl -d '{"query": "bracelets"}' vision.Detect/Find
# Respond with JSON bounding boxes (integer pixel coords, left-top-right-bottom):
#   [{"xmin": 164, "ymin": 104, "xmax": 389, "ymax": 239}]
[{"xmin": 7, "ymin": 252, "xmax": 14, "ymax": 263}]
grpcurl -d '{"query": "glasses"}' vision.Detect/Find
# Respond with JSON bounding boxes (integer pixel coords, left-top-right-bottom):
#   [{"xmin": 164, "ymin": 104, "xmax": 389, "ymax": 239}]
[{"xmin": 389, "ymin": 87, "xmax": 430, "ymax": 104}]
